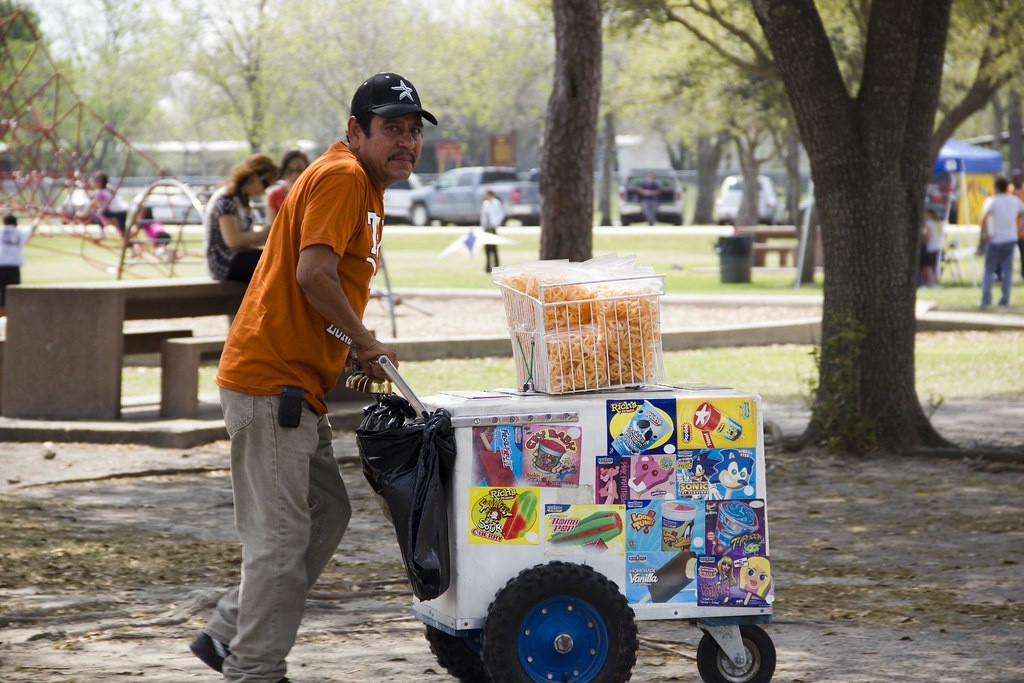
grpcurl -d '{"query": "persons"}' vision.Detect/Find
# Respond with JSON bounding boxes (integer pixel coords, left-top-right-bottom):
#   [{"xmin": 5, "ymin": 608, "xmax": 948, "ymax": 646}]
[
  {"xmin": 0, "ymin": 205, "xmax": 46, "ymax": 308},
  {"xmin": 187, "ymin": 71, "xmax": 439, "ymax": 683},
  {"xmin": 266, "ymin": 150, "xmax": 311, "ymax": 226},
  {"xmin": 978, "ymin": 178, "xmax": 1024, "ymax": 309},
  {"xmin": 637, "ymin": 171, "xmax": 663, "ymax": 226},
  {"xmin": 81, "ymin": 170, "xmax": 138, "ymax": 257},
  {"xmin": 922, "ymin": 207, "xmax": 943, "ymax": 289},
  {"xmin": 479, "ymin": 189, "xmax": 507, "ymax": 273},
  {"xmin": 128, "ymin": 204, "xmax": 183, "ymax": 260},
  {"xmin": 203, "ymin": 153, "xmax": 274, "ymax": 282}
]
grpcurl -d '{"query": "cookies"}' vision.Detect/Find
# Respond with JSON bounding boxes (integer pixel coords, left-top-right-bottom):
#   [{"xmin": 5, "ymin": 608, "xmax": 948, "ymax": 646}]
[{"xmin": 503, "ymin": 274, "xmax": 660, "ymax": 393}]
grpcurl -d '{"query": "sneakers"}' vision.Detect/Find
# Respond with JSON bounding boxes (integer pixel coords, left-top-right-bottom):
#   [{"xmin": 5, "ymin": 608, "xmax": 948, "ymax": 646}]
[{"xmin": 190, "ymin": 632, "xmax": 289, "ymax": 682}]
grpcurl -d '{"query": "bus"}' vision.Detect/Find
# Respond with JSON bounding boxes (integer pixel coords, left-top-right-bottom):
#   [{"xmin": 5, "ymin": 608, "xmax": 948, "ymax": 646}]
[{"xmin": 0, "ymin": 138, "xmax": 318, "ymax": 224}]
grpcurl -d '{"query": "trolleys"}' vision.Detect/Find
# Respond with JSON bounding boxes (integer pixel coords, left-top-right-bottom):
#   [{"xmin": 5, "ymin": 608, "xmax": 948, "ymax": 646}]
[{"xmin": 345, "ymin": 354, "xmax": 779, "ymax": 683}]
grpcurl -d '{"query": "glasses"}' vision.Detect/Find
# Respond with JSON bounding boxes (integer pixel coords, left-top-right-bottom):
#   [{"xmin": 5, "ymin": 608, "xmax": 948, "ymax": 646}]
[
  {"xmin": 256, "ymin": 171, "xmax": 270, "ymax": 188},
  {"xmin": 285, "ymin": 165, "xmax": 304, "ymax": 173}
]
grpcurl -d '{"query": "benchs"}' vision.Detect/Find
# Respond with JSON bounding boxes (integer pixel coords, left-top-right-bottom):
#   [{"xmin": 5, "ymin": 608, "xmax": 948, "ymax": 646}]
[
  {"xmin": 122, "ymin": 329, "xmax": 191, "ymax": 352},
  {"xmin": 755, "ymin": 244, "xmax": 799, "ymax": 265},
  {"xmin": 161, "ymin": 338, "xmax": 228, "ymax": 419}
]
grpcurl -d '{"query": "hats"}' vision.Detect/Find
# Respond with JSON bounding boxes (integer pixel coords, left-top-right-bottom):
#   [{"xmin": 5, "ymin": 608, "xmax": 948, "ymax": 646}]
[{"xmin": 351, "ymin": 71, "xmax": 438, "ymax": 125}]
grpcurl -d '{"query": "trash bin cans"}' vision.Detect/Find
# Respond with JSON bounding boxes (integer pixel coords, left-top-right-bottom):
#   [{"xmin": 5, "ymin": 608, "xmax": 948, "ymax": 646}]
[{"xmin": 717, "ymin": 235, "xmax": 754, "ymax": 284}]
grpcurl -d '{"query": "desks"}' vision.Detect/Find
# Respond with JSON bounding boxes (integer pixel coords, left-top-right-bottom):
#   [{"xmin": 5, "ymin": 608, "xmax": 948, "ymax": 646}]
[
  {"xmin": 4, "ymin": 281, "xmax": 234, "ymax": 422},
  {"xmin": 733, "ymin": 226, "xmax": 824, "ymax": 267}
]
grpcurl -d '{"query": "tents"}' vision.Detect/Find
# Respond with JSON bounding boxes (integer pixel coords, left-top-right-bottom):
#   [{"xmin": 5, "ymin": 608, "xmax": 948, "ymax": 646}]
[{"xmin": 794, "ymin": 137, "xmax": 1001, "ymax": 289}]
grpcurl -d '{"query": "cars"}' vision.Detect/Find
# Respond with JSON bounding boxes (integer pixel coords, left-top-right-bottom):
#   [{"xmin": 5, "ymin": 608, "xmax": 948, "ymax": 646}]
[
  {"xmin": 407, "ymin": 167, "xmax": 542, "ymax": 230},
  {"xmin": 621, "ymin": 165, "xmax": 689, "ymax": 228},
  {"xmin": 711, "ymin": 173, "xmax": 783, "ymax": 227},
  {"xmin": 378, "ymin": 171, "xmax": 420, "ymax": 221}
]
